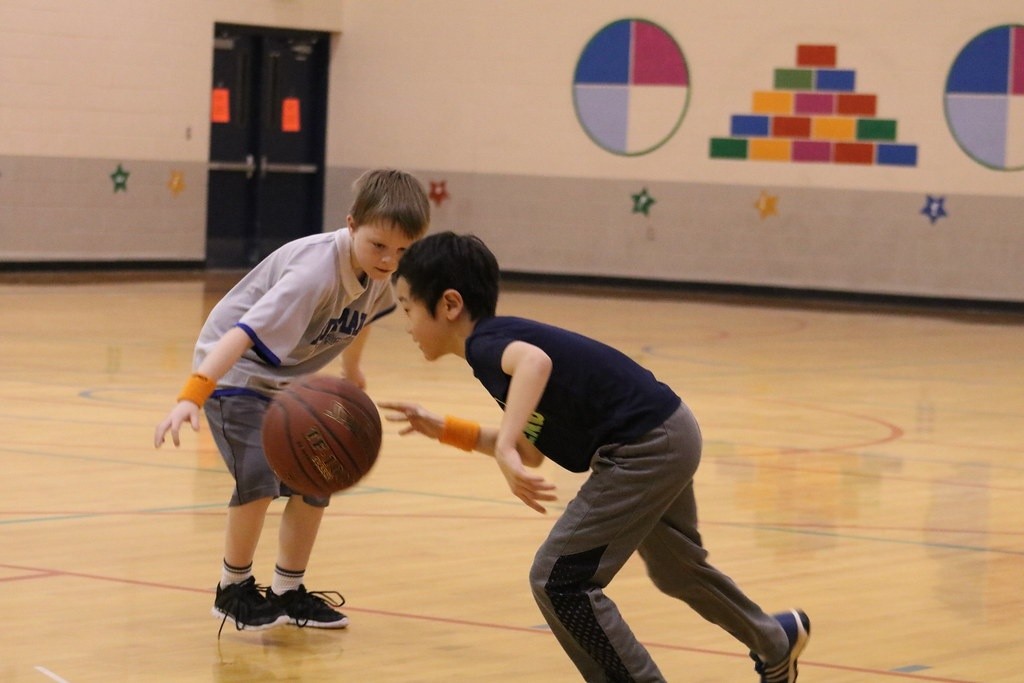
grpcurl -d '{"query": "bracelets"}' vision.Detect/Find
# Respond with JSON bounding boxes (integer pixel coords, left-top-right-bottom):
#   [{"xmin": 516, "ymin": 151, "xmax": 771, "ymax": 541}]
[
  {"xmin": 438, "ymin": 414, "xmax": 480, "ymax": 452},
  {"xmin": 178, "ymin": 372, "xmax": 214, "ymax": 409}
]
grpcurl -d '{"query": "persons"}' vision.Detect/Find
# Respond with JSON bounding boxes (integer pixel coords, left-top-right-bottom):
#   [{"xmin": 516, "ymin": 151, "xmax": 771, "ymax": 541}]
[
  {"xmin": 378, "ymin": 231, "xmax": 811, "ymax": 683},
  {"xmin": 154, "ymin": 167, "xmax": 431, "ymax": 640}
]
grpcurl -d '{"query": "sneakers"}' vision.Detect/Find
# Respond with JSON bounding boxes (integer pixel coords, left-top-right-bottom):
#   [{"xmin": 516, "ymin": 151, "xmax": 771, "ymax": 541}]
[
  {"xmin": 265, "ymin": 584, "xmax": 349, "ymax": 629},
  {"xmin": 211, "ymin": 575, "xmax": 290, "ymax": 631},
  {"xmin": 749, "ymin": 608, "xmax": 812, "ymax": 683}
]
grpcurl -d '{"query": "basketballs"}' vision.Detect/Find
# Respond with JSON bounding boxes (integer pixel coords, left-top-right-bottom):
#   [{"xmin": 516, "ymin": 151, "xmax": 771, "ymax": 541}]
[{"xmin": 259, "ymin": 373, "xmax": 383, "ymax": 499}]
